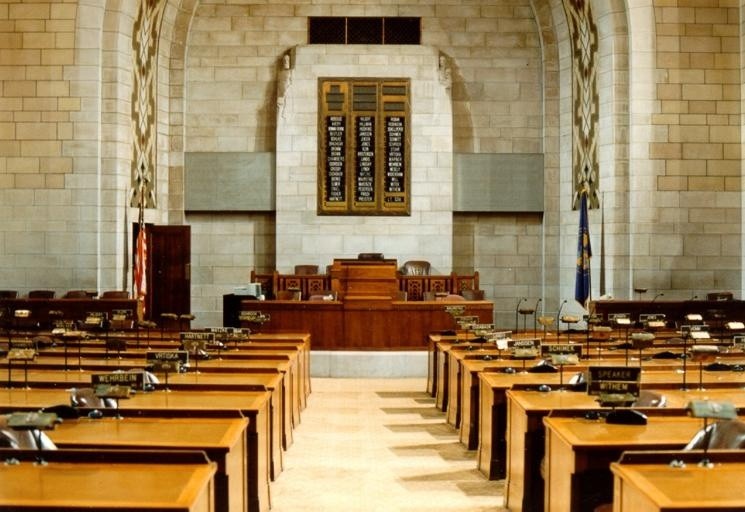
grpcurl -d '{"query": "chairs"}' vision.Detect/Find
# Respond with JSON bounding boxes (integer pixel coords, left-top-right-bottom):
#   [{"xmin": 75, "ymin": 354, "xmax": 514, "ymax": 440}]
[
  {"xmin": 404, "ymin": 260, "xmax": 431, "ymax": 275},
  {"xmin": 396, "ymin": 291, "xmax": 407, "ymax": 301},
  {"xmin": 707, "ymin": 292, "xmax": 733, "ymax": 301},
  {"xmin": 358, "ymin": 253, "xmax": 385, "ymax": 261},
  {"xmin": 311, "ymin": 289, "xmax": 338, "ymax": 301},
  {"xmin": 295, "ymin": 265, "xmax": 319, "ymax": 276},
  {"xmin": 275, "ymin": 287, "xmax": 302, "ymax": 300},
  {"xmin": 0, "ymin": 290, "xmax": 128, "ymax": 299},
  {"xmin": 424, "ymin": 290, "xmax": 485, "ymax": 301}
]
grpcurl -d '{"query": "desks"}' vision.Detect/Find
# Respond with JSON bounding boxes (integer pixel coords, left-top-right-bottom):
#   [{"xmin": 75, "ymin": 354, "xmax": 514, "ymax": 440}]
[
  {"xmin": 0, "ymin": 319, "xmax": 312, "ymax": 512},
  {"xmin": 593, "ymin": 299, "xmax": 745, "ymax": 330},
  {"xmin": 427, "ymin": 328, "xmax": 745, "ymax": 512},
  {"xmin": 0, "ymin": 298, "xmax": 137, "ymax": 333}
]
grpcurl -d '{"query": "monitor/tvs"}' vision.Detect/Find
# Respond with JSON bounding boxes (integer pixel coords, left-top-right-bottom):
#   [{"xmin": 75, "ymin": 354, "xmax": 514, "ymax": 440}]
[{"xmin": 247, "ymin": 283, "xmax": 261, "ymax": 299}]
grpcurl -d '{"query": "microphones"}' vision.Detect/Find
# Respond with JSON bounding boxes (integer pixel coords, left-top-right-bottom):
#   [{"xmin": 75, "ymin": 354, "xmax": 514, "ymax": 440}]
[{"xmin": 518, "ymin": 287, "xmax": 741, "ymax": 389}]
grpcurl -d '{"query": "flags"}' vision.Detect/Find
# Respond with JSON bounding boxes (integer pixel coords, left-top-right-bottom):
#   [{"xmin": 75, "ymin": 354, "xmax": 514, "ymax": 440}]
[
  {"xmin": 573, "ymin": 187, "xmax": 593, "ymax": 309},
  {"xmin": 133, "ymin": 214, "xmax": 149, "ymax": 315}
]
